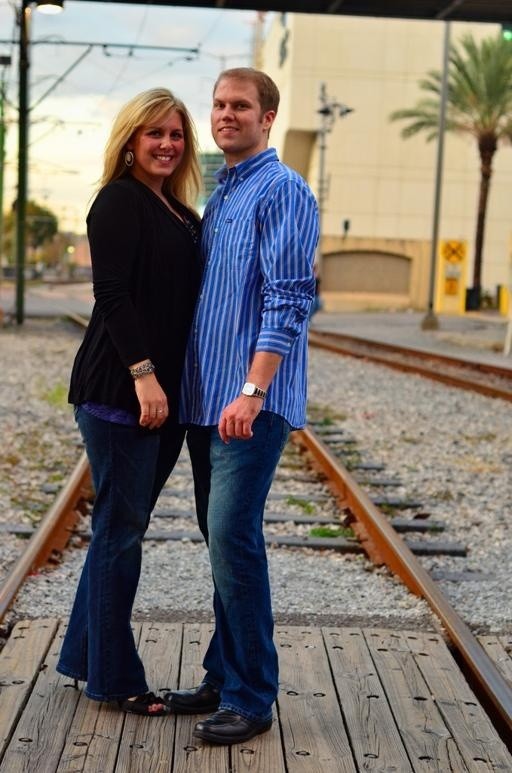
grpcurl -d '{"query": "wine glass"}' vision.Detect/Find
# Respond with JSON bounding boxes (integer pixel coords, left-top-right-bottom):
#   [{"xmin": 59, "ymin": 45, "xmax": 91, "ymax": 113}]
[{"xmin": 158, "ymin": 407, "xmax": 166, "ymax": 414}]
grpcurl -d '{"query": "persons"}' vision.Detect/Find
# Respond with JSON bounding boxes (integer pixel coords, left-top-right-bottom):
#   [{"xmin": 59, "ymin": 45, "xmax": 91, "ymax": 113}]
[
  {"xmin": 157, "ymin": 67, "xmax": 319, "ymax": 743},
  {"xmin": 52, "ymin": 88, "xmax": 202, "ymax": 718}
]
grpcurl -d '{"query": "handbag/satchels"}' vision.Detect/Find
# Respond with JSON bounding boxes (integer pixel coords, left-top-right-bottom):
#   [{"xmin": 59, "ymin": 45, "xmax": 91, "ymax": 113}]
[{"xmin": 125, "ymin": 358, "xmax": 158, "ymax": 383}]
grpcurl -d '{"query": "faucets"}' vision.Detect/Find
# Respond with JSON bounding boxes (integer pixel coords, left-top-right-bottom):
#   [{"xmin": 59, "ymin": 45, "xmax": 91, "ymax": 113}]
[{"xmin": 116, "ymin": 690, "xmax": 166, "ymax": 717}]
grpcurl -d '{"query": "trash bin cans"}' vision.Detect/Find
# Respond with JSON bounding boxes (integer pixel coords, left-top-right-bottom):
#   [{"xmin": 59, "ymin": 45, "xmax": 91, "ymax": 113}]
[{"xmin": 239, "ymin": 379, "xmax": 269, "ymax": 401}]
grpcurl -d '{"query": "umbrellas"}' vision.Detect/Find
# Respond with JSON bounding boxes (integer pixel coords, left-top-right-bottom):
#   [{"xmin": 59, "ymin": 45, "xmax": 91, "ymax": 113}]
[
  {"xmin": 163, "ymin": 682, "xmax": 220, "ymax": 713},
  {"xmin": 191, "ymin": 707, "xmax": 273, "ymax": 746}
]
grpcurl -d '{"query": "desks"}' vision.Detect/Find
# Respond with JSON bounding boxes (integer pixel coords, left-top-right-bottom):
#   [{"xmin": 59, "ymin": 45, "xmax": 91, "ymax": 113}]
[
  {"xmin": 13, "ymin": 0, "xmax": 67, "ymax": 328},
  {"xmin": 316, "ymin": 94, "xmax": 354, "ymax": 208}
]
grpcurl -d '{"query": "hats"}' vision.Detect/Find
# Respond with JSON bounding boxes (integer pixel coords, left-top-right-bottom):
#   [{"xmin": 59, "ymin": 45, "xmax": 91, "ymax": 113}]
[{"xmin": 21, "ymin": 259, "xmax": 93, "ymax": 283}]
